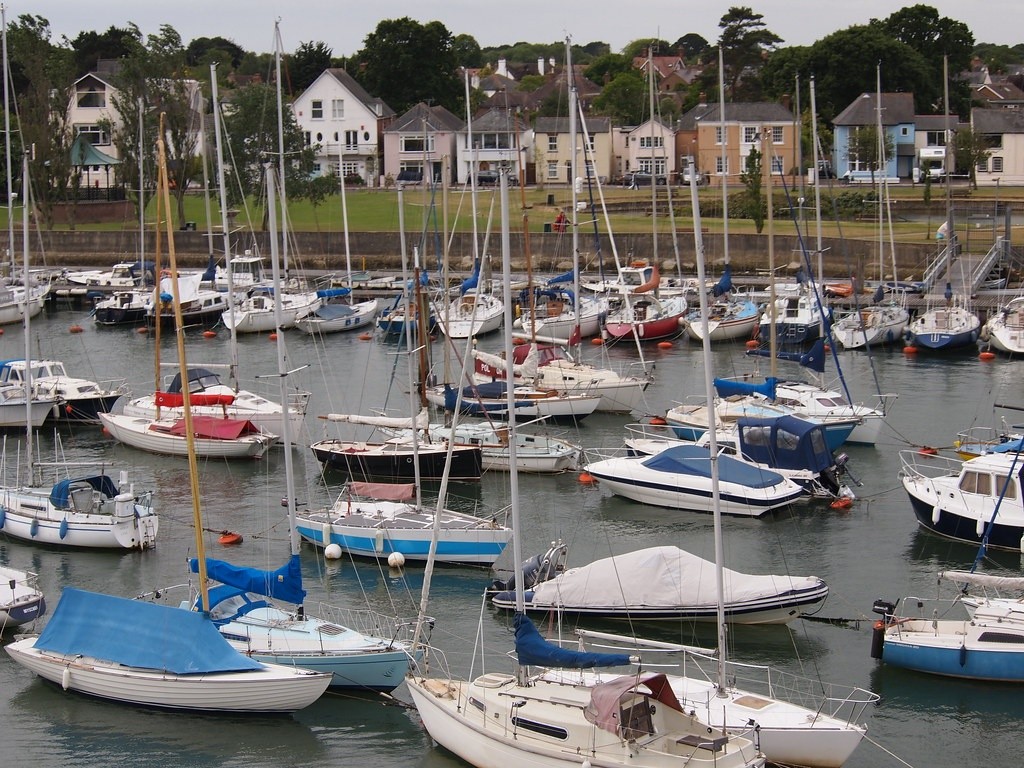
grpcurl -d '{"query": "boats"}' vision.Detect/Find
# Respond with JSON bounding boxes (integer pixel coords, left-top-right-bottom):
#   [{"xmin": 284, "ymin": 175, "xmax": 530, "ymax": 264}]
[{"xmin": 2, "ymin": 37, "xmax": 1023, "ymax": 768}]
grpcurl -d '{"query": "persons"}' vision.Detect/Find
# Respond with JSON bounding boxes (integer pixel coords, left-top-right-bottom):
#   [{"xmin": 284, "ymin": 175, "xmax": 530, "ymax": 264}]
[
  {"xmin": 553, "ymin": 212, "xmax": 572, "ymax": 233},
  {"xmin": 841, "ymin": 170, "xmax": 853, "ymax": 179},
  {"xmin": 935, "ymin": 220, "xmax": 948, "ymax": 244},
  {"xmin": 628, "ymin": 170, "xmax": 641, "ymax": 190}
]
